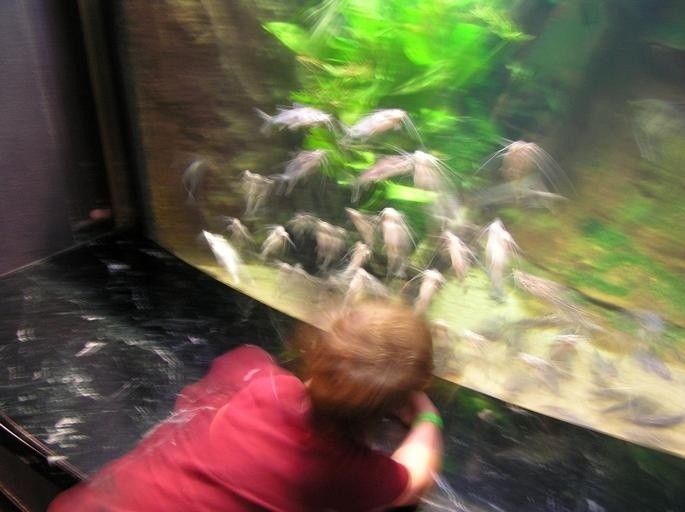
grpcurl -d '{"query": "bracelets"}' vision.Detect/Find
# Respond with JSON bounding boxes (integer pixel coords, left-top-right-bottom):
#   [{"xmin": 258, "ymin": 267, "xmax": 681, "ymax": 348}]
[{"xmin": 410, "ymin": 412, "xmax": 447, "ymax": 428}]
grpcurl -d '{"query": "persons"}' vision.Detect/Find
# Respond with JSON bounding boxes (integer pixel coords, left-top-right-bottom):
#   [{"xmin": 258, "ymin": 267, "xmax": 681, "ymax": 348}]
[{"xmin": 46, "ymin": 293, "xmax": 445, "ymax": 511}]
